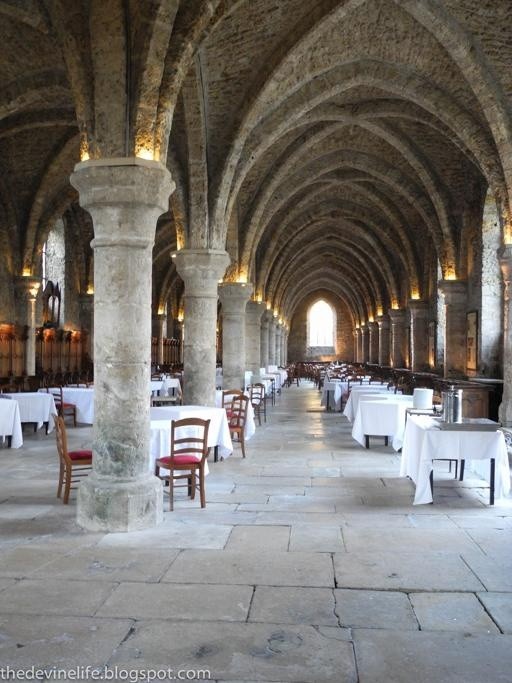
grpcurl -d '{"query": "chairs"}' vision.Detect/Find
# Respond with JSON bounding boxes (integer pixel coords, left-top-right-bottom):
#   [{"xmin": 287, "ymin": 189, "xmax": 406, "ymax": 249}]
[
  {"xmin": 51, "ymin": 412, "xmax": 93, "ymax": 504},
  {"xmin": 154, "ymin": 417, "xmax": 211, "ymax": 512},
  {"xmin": 216, "ymin": 383, "xmax": 263, "ymax": 459},
  {"xmin": 245, "ymin": 360, "xmax": 409, "ymax": 409}
]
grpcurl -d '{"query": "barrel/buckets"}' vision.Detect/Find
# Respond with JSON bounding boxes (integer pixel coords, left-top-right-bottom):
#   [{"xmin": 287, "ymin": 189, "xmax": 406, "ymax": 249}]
[{"xmin": 440, "ymin": 389, "xmax": 464, "ymax": 425}]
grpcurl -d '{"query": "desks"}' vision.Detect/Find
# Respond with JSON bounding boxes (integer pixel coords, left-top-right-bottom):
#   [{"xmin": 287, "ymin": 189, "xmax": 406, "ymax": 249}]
[
  {"xmin": 351, "ymin": 389, "xmax": 414, "ymax": 449},
  {"xmin": 0, "ymin": 383, "xmax": 94, "ymax": 449},
  {"xmin": 151, "ymin": 372, "xmax": 183, "ymax": 406},
  {"xmin": 149, "ymin": 406, "xmax": 225, "ymax": 497},
  {"xmin": 406, "ymin": 413, "xmax": 504, "ymax": 506}
]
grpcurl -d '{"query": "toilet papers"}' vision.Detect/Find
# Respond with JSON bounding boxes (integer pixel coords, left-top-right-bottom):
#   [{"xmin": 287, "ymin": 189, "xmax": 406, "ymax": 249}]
[{"xmin": 412, "ymin": 388, "xmax": 433, "ymax": 410}]
